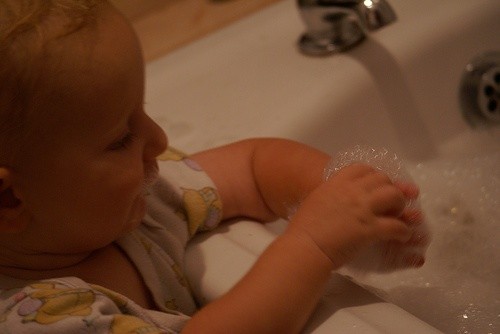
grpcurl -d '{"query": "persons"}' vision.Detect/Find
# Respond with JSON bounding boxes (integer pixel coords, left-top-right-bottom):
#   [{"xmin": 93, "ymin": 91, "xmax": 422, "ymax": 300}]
[{"xmin": 0, "ymin": 0, "xmax": 431, "ymax": 333}]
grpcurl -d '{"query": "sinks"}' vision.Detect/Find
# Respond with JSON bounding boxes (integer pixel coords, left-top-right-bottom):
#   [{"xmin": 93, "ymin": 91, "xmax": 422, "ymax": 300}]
[{"xmin": 232, "ymin": 1, "xmax": 500, "ymax": 334}]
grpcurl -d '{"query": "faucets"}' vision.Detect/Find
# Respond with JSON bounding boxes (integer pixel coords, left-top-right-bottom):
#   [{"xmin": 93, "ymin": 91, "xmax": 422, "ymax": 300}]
[{"xmin": 295, "ymin": 0, "xmax": 398, "ymax": 45}]
